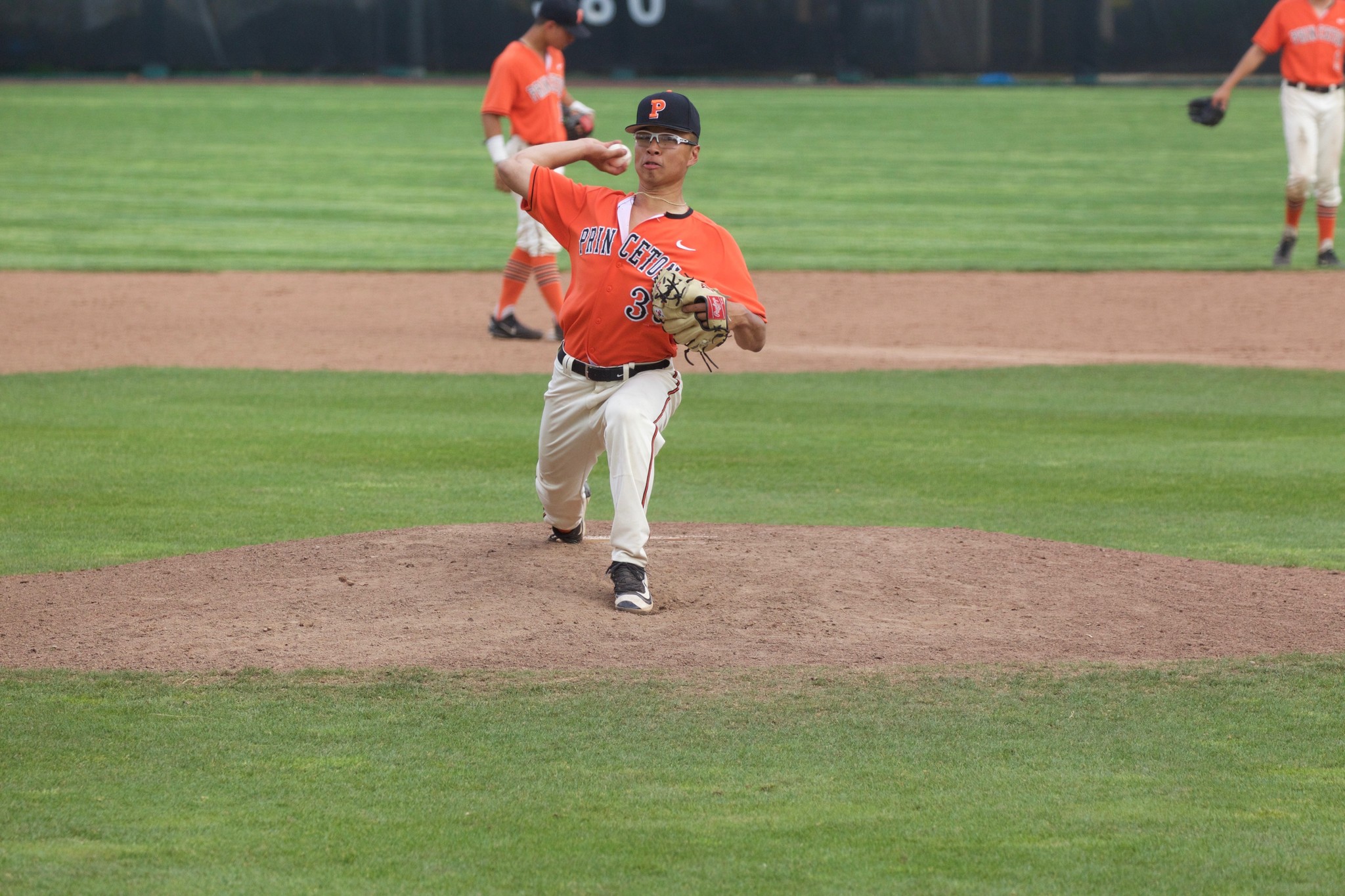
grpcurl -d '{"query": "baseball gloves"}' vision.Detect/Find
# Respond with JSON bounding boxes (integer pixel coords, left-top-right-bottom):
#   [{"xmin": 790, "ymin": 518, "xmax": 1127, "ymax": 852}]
[
  {"xmin": 564, "ymin": 106, "xmax": 593, "ymax": 140},
  {"xmin": 1189, "ymin": 96, "xmax": 1223, "ymax": 126},
  {"xmin": 653, "ymin": 269, "xmax": 726, "ymax": 351}
]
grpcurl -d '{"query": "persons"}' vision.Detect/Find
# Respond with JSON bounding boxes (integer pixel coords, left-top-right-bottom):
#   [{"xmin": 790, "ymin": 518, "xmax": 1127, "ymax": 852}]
[
  {"xmin": 1212, "ymin": 0, "xmax": 1345, "ymax": 265},
  {"xmin": 480, "ymin": 0, "xmax": 593, "ymax": 340},
  {"xmin": 500, "ymin": 89, "xmax": 769, "ymax": 612}
]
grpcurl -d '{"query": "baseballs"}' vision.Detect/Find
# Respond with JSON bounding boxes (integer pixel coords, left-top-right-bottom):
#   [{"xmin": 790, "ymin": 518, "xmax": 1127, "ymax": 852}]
[{"xmin": 609, "ymin": 144, "xmax": 633, "ymax": 168}]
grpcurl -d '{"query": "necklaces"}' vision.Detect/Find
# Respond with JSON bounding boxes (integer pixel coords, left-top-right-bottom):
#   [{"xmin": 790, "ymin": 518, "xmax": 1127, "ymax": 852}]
[{"xmin": 630, "ymin": 192, "xmax": 686, "ymax": 207}]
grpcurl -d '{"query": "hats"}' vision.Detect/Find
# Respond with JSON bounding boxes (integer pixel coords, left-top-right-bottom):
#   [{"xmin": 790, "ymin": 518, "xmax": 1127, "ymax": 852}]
[
  {"xmin": 625, "ymin": 90, "xmax": 701, "ymax": 138},
  {"xmin": 540, "ymin": 0, "xmax": 591, "ymax": 38}
]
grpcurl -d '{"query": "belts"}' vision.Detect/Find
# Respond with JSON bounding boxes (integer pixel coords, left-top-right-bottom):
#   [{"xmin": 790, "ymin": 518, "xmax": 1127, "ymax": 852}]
[
  {"xmin": 1287, "ymin": 81, "xmax": 1343, "ymax": 93},
  {"xmin": 557, "ymin": 341, "xmax": 670, "ymax": 382}
]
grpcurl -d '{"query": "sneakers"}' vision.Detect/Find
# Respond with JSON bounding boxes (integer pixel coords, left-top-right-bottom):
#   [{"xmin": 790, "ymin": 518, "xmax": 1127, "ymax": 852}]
[
  {"xmin": 605, "ymin": 560, "xmax": 653, "ymax": 615},
  {"xmin": 549, "ymin": 481, "xmax": 591, "ymax": 545}
]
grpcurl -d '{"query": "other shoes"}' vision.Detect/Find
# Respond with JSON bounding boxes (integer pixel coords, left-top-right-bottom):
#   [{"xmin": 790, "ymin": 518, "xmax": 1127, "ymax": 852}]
[
  {"xmin": 488, "ymin": 311, "xmax": 542, "ymax": 339},
  {"xmin": 555, "ymin": 324, "xmax": 564, "ymax": 340},
  {"xmin": 1318, "ymin": 250, "xmax": 1341, "ymax": 268},
  {"xmin": 1274, "ymin": 232, "xmax": 1297, "ymax": 266}
]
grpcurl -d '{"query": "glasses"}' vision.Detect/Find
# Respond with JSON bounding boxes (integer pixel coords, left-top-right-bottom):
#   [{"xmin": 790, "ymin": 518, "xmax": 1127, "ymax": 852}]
[{"xmin": 633, "ymin": 130, "xmax": 698, "ymax": 149}]
